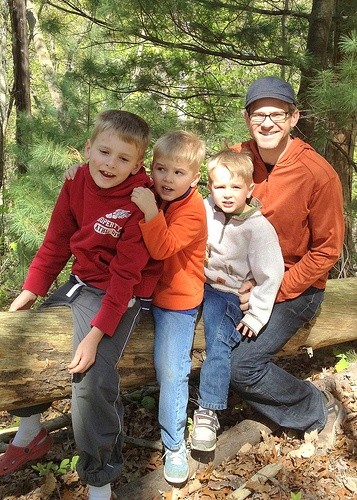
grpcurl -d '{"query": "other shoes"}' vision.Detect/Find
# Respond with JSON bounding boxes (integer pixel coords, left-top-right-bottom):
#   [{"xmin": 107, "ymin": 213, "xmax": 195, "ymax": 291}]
[{"xmin": 318, "ymin": 390, "xmax": 345, "ymax": 454}]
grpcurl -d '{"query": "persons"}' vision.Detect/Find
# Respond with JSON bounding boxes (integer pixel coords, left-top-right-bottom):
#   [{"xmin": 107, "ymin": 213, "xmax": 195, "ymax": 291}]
[
  {"xmin": 0, "ymin": 109, "xmax": 160, "ymax": 500},
  {"xmin": 128, "ymin": 130, "xmax": 209, "ymax": 482},
  {"xmin": 189, "ymin": 150, "xmax": 285, "ymax": 451},
  {"xmin": 221, "ymin": 76, "xmax": 345, "ymax": 452}
]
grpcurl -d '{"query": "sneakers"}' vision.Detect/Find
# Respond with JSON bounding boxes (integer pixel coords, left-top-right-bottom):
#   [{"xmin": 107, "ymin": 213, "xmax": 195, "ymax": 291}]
[
  {"xmin": 191, "ymin": 409, "xmax": 221, "ymax": 452},
  {"xmin": 160, "ymin": 443, "xmax": 189, "ymax": 483}
]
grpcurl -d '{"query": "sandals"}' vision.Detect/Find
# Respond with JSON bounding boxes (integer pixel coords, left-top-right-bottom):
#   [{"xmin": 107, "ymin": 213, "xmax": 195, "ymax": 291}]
[{"xmin": 0, "ymin": 429, "xmax": 53, "ymax": 476}]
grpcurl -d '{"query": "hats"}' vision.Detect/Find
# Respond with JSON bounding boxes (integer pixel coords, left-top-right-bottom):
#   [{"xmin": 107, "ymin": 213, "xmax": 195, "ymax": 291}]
[{"xmin": 244, "ymin": 75, "xmax": 297, "ymax": 107}]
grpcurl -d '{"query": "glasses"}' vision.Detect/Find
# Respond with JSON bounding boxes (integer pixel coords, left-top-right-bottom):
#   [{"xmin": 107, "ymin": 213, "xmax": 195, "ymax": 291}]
[{"xmin": 248, "ymin": 109, "xmax": 294, "ymax": 122}]
